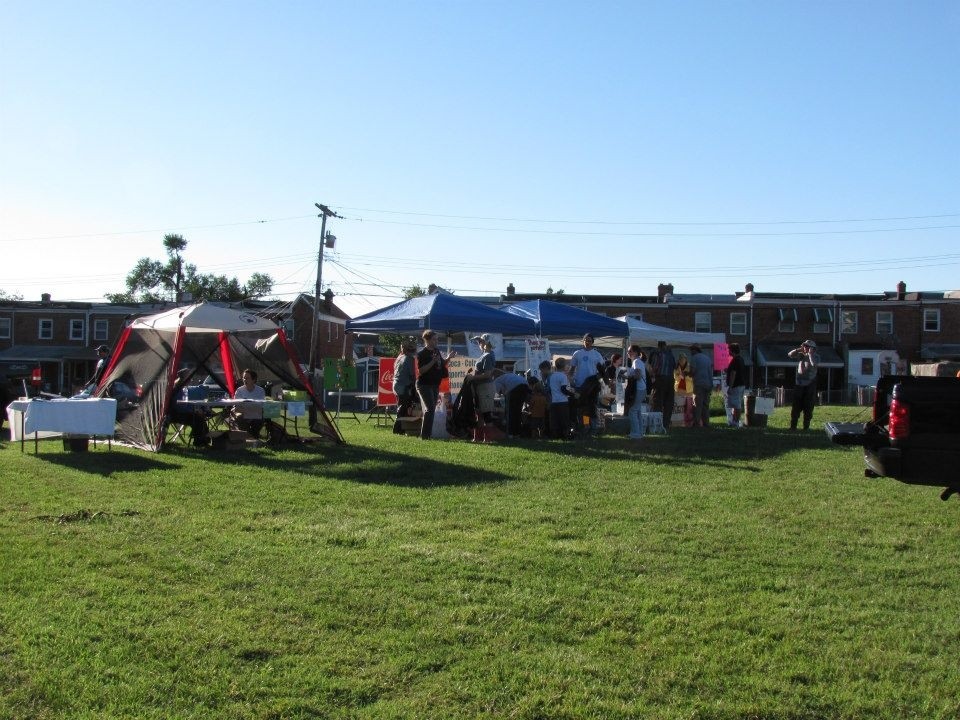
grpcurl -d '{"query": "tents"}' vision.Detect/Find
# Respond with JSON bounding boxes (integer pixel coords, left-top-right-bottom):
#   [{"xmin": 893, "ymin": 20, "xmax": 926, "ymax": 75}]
[
  {"xmin": 612, "ymin": 315, "xmax": 726, "ymax": 393},
  {"xmin": 498, "ymin": 297, "xmax": 630, "ymax": 371},
  {"xmin": 337, "ymin": 291, "xmax": 536, "ymax": 437},
  {"xmin": 91, "ymin": 300, "xmax": 343, "ymax": 452}
]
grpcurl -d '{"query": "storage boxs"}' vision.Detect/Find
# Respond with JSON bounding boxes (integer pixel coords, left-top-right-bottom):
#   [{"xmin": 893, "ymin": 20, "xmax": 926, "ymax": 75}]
[
  {"xmin": 206, "ymin": 429, "xmax": 247, "ymax": 448},
  {"xmin": 62, "ymin": 433, "xmax": 90, "ymax": 451},
  {"xmin": 284, "ymin": 390, "xmax": 307, "ymax": 402},
  {"xmin": 598, "ymin": 403, "xmax": 663, "ymax": 435}
]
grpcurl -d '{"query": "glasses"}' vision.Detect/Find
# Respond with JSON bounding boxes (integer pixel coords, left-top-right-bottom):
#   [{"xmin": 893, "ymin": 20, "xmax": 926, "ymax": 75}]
[{"xmin": 803, "ymin": 344, "xmax": 812, "ymax": 348}]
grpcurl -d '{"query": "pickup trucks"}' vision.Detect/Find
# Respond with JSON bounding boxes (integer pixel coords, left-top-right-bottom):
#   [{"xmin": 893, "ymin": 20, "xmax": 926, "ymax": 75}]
[{"xmin": 824, "ymin": 375, "xmax": 960, "ymax": 501}]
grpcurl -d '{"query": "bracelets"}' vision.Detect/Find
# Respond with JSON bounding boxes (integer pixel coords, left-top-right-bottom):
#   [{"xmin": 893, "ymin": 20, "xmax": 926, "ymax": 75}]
[{"xmin": 796, "ymin": 348, "xmax": 797, "ymax": 352}]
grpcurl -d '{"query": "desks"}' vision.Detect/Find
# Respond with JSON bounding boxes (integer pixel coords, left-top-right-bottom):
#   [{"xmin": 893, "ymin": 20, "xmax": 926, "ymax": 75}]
[
  {"xmin": 5, "ymin": 398, "xmax": 117, "ymax": 455},
  {"xmin": 171, "ymin": 399, "xmax": 313, "ymax": 448},
  {"xmin": 328, "ymin": 391, "xmax": 395, "ymax": 424}
]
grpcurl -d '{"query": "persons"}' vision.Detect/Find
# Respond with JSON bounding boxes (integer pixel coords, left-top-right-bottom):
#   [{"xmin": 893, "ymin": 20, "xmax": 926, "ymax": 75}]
[
  {"xmin": 649, "ymin": 341, "xmax": 677, "ymax": 427},
  {"xmin": 80, "ymin": 344, "xmax": 109, "ymax": 391},
  {"xmin": 172, "ymin": 367, "xmax": 211, "ymax": 445},
  {"xmin": 415, "ymin": 329, "xmax": 458, "ymax": 440},
  {"xmin": 496, "ymin": 333, "xmax": 695, "ymax": 437},
  {"xmin": 549, "ymin": 358, "xmax": 575, "ymax": 438},
  {"xmin": 622, "ymin": 345, "xmax": 648, "ymax": 439},
  {"xmin": 392, "ymin": 340, "xmax": 414, "ymax": 435},
  {"xmin": 787, "ymin": 339, "xmax": 822, "ymax": 432},
  {"xmin": 688, "ymin": 344, "xmax": 713, "ymax": 429},
  {"xmin": 233, "ymin": 368, "xmax": 266, "ymax": 439},
  {"xmin": 724, "ymin": 342, "xmax": 747, "ymax": 427},
  {"xmin": 467, "ymin": 333, "xmax": 497, "ymax": 443}
]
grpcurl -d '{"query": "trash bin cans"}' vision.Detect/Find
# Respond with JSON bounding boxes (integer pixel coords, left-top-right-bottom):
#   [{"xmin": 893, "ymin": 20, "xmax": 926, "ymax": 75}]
[
  {"xmin": 743, "ymin": 395, "xmax": 769, "ymax": 428},
  {"xmin": 182, "ymin": 386, "xmax": 209, "ymax": 401}
]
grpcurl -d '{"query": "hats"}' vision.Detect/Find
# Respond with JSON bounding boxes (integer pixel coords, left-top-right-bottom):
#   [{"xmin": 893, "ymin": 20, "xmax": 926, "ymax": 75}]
[
  {"xmin": 177, "ymin": 368, "xmax": 196, "ymax": 383},
  {"xmin": 688, "ymin": 343, "xmax": 704, "ymax": 353},
  {"xmin": 469, "ymin": 333, "xmax": 497, "ymax": 349},
  {"xmin": 93, "ymin": 344, "xmax": 109, "ymax": 352},
  {"xmin": 800, "ymin": 340, "xmax": 816, "ymax": 348}
]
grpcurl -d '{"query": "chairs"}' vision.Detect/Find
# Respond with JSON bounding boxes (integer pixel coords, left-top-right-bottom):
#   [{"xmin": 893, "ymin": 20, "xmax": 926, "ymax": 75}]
[{"xmin": 165, "ymin": 380, "xmax": 273, "ymax": 445}]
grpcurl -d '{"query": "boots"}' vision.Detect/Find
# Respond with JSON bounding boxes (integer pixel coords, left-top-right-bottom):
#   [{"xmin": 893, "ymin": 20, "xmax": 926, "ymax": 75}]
[{"xmin": 471, "ymin": 425, "xmax": 495, "ymax": 443}]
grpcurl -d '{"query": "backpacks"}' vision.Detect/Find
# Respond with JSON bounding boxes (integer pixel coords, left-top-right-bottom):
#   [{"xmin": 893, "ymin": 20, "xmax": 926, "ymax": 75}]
[{"xmin": 637, "ymin": 359, "xmax": 652, "ymax": 395}]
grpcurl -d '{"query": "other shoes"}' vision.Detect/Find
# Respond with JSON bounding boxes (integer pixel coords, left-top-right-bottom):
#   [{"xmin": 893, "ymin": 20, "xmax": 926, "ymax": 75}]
[
  {"xmin": 192, "ymin": 441, "xmax": 209, "ymax": 447},
  {"xmin": 393, "ymin": 430, "xmax": 405, "ymax": 434},
  {"xmin": 800, "ymin": 429, "xmax": 809, "ymax": 434},
  {"xmin": 787, "ymin": 428, "xmax": 797, "ymax": 433},
  {"xmin": 507, "ymin": 432, "xmax": 521, "ymax": 439}
]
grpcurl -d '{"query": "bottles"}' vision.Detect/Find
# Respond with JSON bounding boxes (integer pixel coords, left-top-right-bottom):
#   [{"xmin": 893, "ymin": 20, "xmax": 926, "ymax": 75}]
[{"xmin": 182, "ymin": 386, "xmax": 188, "ymax": 402}]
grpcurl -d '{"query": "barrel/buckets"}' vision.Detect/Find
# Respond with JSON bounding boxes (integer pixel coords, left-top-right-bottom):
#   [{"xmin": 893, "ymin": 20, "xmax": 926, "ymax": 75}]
[{"xmin": 743, "ymin": 395, "xmax": 769, "ymax": 427}]
